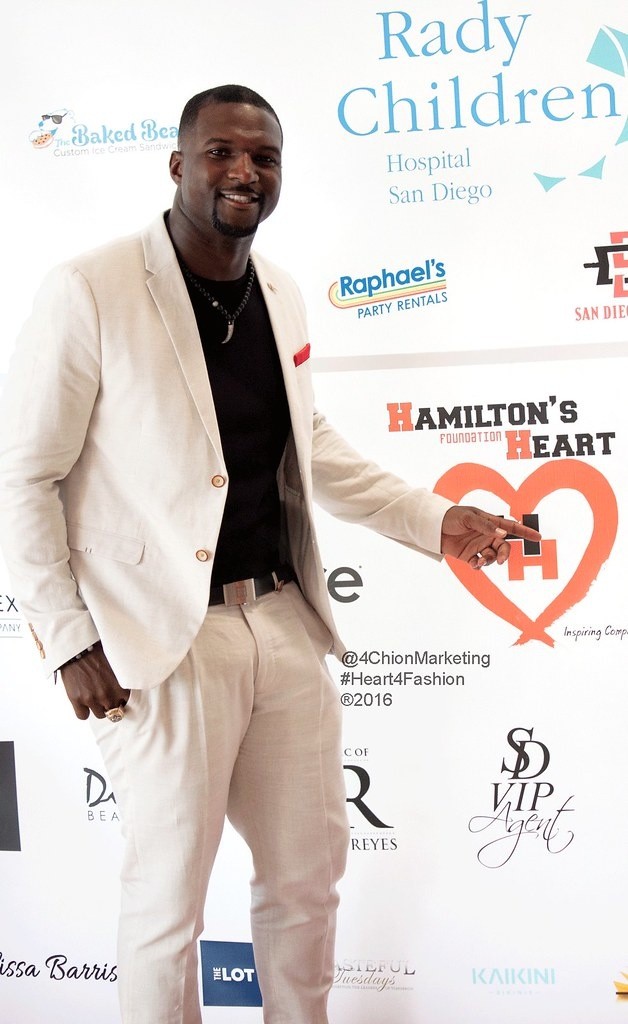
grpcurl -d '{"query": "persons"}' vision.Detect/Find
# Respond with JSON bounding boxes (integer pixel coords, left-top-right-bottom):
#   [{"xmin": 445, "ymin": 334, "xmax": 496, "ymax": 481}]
[{"xmin": 0, "ymin": 83, "xmax": 543, "ymax": 1024}]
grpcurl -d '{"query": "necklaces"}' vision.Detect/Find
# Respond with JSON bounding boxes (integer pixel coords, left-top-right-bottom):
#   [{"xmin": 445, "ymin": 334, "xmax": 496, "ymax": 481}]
[{"xmin": 175, "ymin": 245, "xmax": 255, "ymax": 344}]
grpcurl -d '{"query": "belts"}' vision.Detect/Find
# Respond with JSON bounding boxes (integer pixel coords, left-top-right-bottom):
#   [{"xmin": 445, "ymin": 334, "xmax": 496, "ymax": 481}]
[{"xmin": 210, "ymin": 567, "xmax": 292, "ymax": 606}]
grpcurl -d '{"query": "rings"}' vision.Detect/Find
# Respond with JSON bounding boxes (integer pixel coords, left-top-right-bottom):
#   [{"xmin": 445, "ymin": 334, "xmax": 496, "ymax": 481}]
[{"xmin": 104, "ymin": 704, "xmax": 125, "ymax": 722}]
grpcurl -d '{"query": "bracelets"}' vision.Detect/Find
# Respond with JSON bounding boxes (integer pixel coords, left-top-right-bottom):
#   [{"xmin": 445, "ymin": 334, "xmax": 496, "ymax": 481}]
[{"xmin": 54, "ymin": 645, "xmax": 94, "ymax": 684}]
